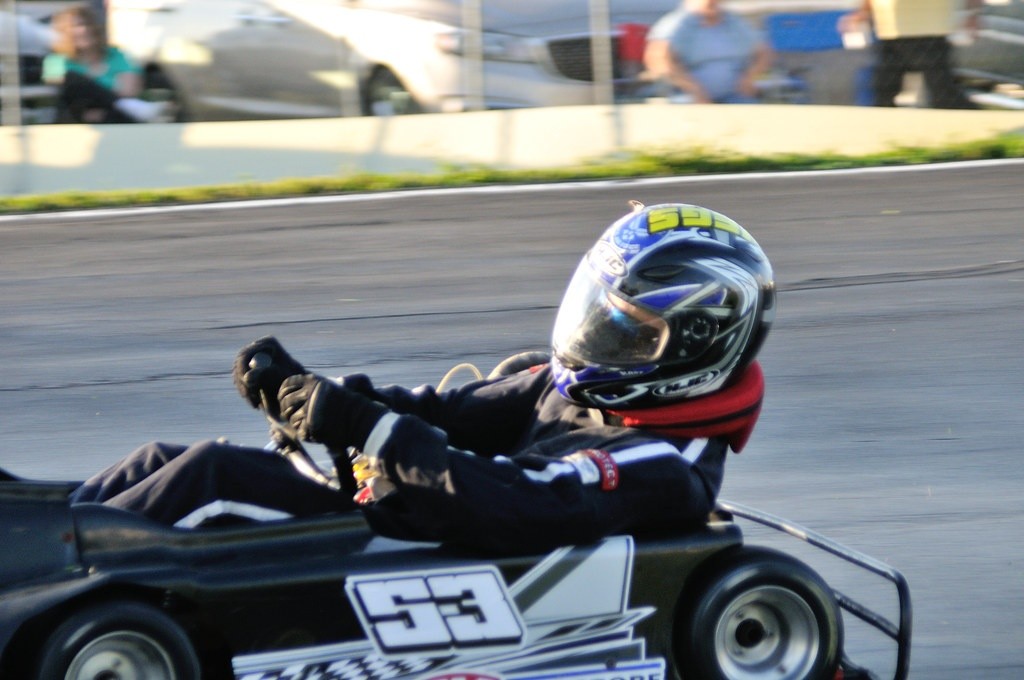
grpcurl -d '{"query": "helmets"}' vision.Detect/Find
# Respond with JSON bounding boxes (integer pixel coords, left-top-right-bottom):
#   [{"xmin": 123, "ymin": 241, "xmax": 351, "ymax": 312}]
[{"xmin": 551, "ymin": 203, "xmax": 778, "ymax": 410}]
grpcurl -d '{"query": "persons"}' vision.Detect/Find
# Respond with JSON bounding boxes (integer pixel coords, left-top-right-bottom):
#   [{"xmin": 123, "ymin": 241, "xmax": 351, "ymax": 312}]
[
  {"xmin": 849, "ymin": 0, "xmax": 984, "ymax": 109},
  {"xmin": 67, "ymin": 203, "xmax": 777, "ymax": 560},
  {"xmin": 42, "ymin": 8, "xmax": 189, "ymax": 123},
  {"xmin": 642, "ymin": 0, "xmax": 772, "ymax": 103}
]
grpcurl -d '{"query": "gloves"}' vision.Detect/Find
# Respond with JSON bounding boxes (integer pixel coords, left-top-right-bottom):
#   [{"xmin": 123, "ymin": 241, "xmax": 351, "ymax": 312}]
[{"xmin": 232, "ymin": 335, "xmax": 392, "ymax": 453}]
